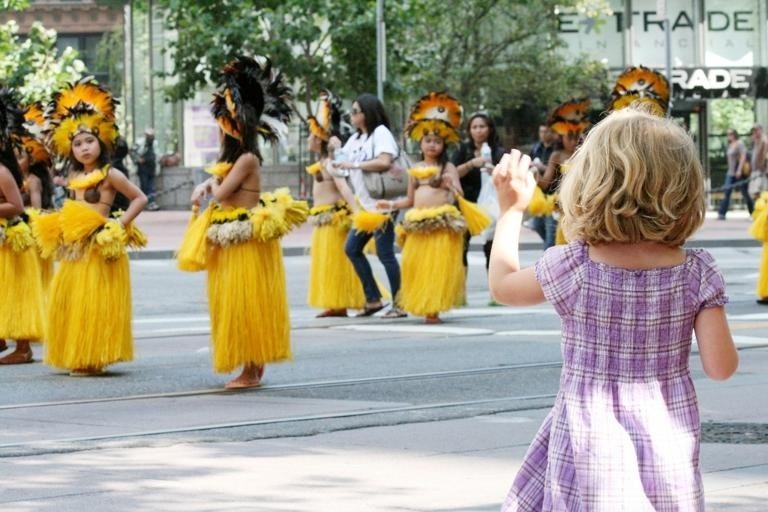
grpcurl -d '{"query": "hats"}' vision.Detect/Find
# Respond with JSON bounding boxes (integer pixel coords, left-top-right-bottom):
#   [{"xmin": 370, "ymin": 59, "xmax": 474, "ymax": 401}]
[
  {"xmin": 549, "ymin": 67, "xmax": 670, "ymax": 135},
  {"xmin": 209, "ymin": 54, "xmax": 292, "ymax": 147},
  {"xmin": 405, "ymin": 92, "xmax": 466, "ymax": 146},
  {"xmin": 308, "ymin": 88, "xmax": 342, "ymax": 143},
  {"xmin": 0, "ymin": 78, "xmax": 120, "ymax": 167}
]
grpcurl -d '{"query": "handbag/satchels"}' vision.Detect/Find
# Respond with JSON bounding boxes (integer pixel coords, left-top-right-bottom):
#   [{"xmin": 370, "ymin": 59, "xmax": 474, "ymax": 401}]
[{"xmin": 362, "ymin": 159, "xmax": 407, "ymax": 199}]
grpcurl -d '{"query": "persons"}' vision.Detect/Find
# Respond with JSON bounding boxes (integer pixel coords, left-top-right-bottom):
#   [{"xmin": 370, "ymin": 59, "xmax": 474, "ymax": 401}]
[
  {"xmin": 526, "ymin": 96, "xmax": 592, "ymax": 254},
  {"xmin": 607, "ymin": 66, "xmax": 673, "ymax": 119},
  {"xmin": 175, "ymin": 52, "xmax": 310, "ymax": 390},
  {"xmin": 1, "ymin": 74, "xmax": 169, "ymax": 377},
  {"xmin": 487, "ymin": 98, "xmax": 739, "ymax": 512},
  {"xmin": 717, "ymin": 123, "xmax": 768, "ymax": 305},
  {"xmin": 303, "ymin": 91, "xmax": 501, "ymax": 325}
]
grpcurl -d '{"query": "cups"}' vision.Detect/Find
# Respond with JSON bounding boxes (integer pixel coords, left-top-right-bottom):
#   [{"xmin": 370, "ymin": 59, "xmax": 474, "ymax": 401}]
[{"xmin": 334, "ymin": 148, "xmax": 348, "ymax": 164}]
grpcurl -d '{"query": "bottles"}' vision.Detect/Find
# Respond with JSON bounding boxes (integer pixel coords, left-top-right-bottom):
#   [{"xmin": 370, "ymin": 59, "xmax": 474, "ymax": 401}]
[{"xmin": 480, "ymin": 141, "xmax": 493, "ymax": 164}]
